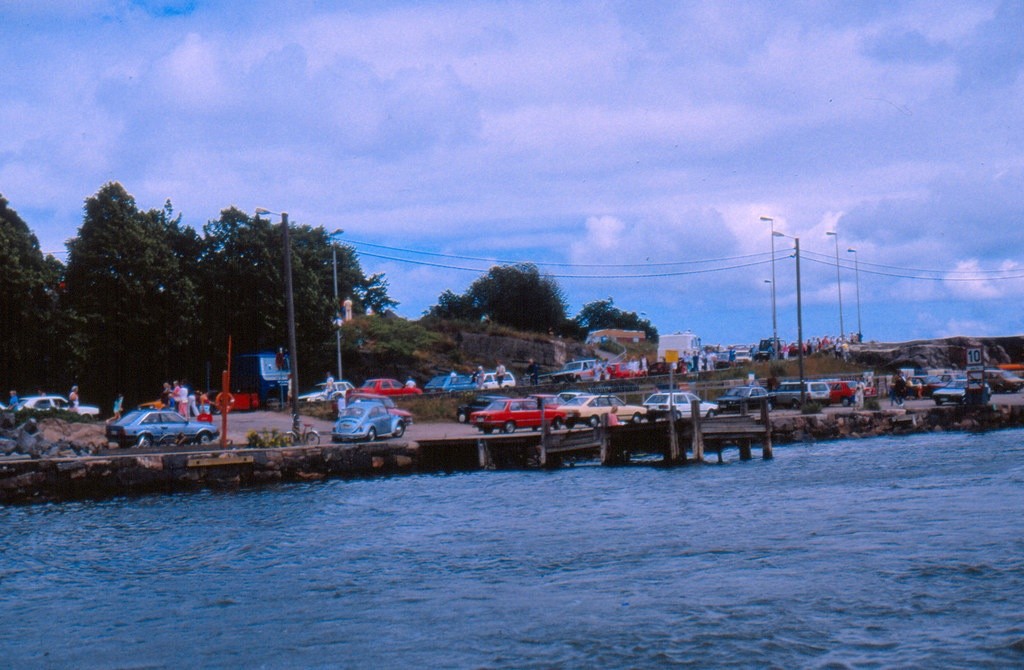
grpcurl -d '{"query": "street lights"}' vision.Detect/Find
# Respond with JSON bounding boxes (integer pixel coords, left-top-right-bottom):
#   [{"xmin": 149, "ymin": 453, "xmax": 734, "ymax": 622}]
[
  {"xmin": 774, "ymin": 232, "xmax": 807, "ymax": 415},
  {"xmin": 827, "ymin": 230, "xmax": 846, "ymax": 340},
  {"xmin": 758, "ymin": 216, "xmax": 779, "ymax": 360},
  {"xmin": 764, "ymin": 279, "xmax": 773, "ymax": 312},
  {"xmin": 255, "ymin": 207, "xmax": 300, "ymax": 443},
  {"xmin": 847, "ymin": 249, "xmax": 862, "ymax": 339},
  {"xmin": 328, "ymin": 228, "xmax": 345, "ymax": 381}
]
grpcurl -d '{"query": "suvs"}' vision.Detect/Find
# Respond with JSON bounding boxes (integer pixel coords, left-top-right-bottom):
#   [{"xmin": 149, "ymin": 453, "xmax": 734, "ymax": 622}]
[
  {"xmin": 769, "ymin": 378, "xmax": 877, "ymax": 410},
  {"xmin": 551, "ymin": 359, "xmax": 688, "ymax": 383}
]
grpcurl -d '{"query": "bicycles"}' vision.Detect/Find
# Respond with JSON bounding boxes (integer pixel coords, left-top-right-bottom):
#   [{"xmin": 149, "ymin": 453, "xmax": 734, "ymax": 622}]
[{"xmin": 281, "ymin": 413, "xmax": 321, "ymax": 445}]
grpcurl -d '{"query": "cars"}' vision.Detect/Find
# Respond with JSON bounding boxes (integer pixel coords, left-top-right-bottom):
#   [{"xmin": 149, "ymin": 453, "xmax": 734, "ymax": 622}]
[
  {"xmin": 423, "ymin": 375, "xmax": 480, "ymax": 392},
  {"xmin": 456, "ymin": 391, "xmax": 650, "ymax": 435},
  {"xmin": 713, "ymin": 386, "xmax": 774, "ymax": 415},
  {"xmin": 735, "ymin": 350, "xmax": 753, "ymax": 364},
  {"xmin": 106, "ymin": 409, "xmax": 220, "ymax": 449},
  {"xmin": 643, "ymin": 391, "xmax": 720, "ymax": 419},
  {"xmin": 13, "ymin": 395, "xmax": 101, "ymax": 423},
  {"xmin": 788, "ymin": 341, "xmax": 808, "ymax": 357},
  {"xmin": 298, "ymin": 379, "xmax": 424, "ymax": 442},
  {"xmin": 141, "ymin": 398, "xmax": 165, "ymax": 412},
  {"xmin": 909, "ymin": 366, "xmax": 1024, "ymax": 406}
]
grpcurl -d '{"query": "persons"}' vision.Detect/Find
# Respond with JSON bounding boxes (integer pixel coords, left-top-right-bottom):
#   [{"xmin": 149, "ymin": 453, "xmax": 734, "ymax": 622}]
[
  {"xmin": 405, "ymin": 376, "xmax": 416, "ymax": 388},
  {"xmin": 6, "ymin": 390, "xmax": 18, "ymax": 411},
  {"xmin": 744, "ymin": 372, "xmax": 779, "ymax": 408},
  {"xmin": 471, "ymin": 366, "xmax": 488, "ymax": 390},
  {"xmin": 106, "ymin": 389, "xmax": 125, "ymax": 423},
  {"xmin": 324, "ymin": 371, "xmax": 335, "ymax": 401},
  {"xmin": 849, "ymin": 370, "xmax": 923, "ymax": 411},
  {"xmin": 493, "ymin": 360, "xmax": 505, "ymax": 388},
  {"xmin": 69, "ymin": 386, "xmax": 79, "ymax": 415},
  {"xmin": 593, "ymin": 357, "xmax": 612, "ymax": 382},
  {"xmin": 728, "ymin": 345, "xmax": 737, "ymax": 369},
  {"xmin": 38, "ymin": 388, "xmax": 46, "ymax": 398},
  {"xmin": 526, "ymin": 358, "xmax": 538, "ymax": 386},
  {"xmin": 671, "ymin": 348, "xmax": 717, "ymax": 374},
  {"xmin": 750, "ymin": 344, "xmax": 759, "ymax": 362},
  {"xmin": 334, "ymin": 392, "xmax": 345, "ymax": 432},
  {"xmin": 608, "ymin": 405, "xmax": 625, "ymax": 426},
  {"xmin": 629, "ymin": 353, "xmax": 650, "ymax": 377},
  {"xmin": 768, "ymin": 331, "xmax": 863, "ymax": 360},
  {"xmin": 161, "ymin": 379, "xmax": 210, "ymax": 421},
  {"xmin": 344, "ymin": 296, "xmax": 352, "ymax": 322}
]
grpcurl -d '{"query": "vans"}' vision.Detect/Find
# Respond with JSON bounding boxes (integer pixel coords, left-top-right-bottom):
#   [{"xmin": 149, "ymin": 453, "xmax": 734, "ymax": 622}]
[
  {"xmin": 756, "ymin": 338, "xmax": 786, "ymax": 361},
  {"xmin": 231, "ymin": 353, "xmax": 292, "ymax": 407},
  {"xmin": 468, "ymin": 371, "xmax": 516, "ymax": 390}
]
